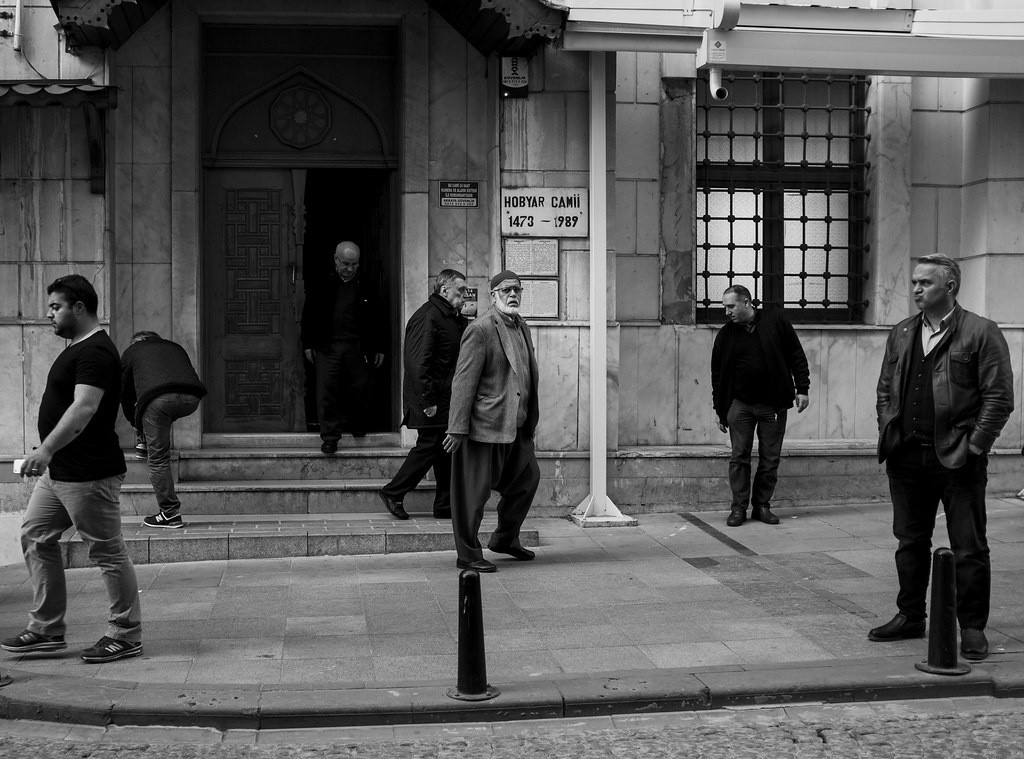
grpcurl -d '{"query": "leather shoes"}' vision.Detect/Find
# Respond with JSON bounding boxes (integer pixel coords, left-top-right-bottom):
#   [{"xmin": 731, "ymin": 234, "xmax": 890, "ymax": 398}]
[
  {"xmin": 727, "ymin": 509, "xmax": 746, "ymax": 526},
  {"xmin": 960, "ymin": 627, "xmax": 989, "ymax": 659},
  {"xmin": 457, "ymin": 557, "xmax": 497, "ymax": 572},
  {"xmin": 868, "ymin": 614, "xmax": 926, "ymax": 641},
  {"xmin": 434, "ymin": 510, "xmax": 451, "ymax": 519},
  {"xmin": 751, "ymin": 507, "xmax": 779, "ymax": 524},
  {"xmin": 379, "ymin": 490, "xmax": 409, "ymax": 520},
  {"xmin": 321, "ymin": 440, "xmax": 337, "ymax": 453},
  {"xmin": 352, "ymin": 427, "xmax": 365, "ymax": 437},
  {"xmin": 488, "ymin": 539, "xmax": 535, "ymax": 560}
]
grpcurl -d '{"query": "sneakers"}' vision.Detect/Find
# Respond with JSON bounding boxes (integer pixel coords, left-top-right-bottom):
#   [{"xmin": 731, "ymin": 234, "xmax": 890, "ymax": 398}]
[
  {"xmin": 1, "ymin": 629, "xmax": 67, "ymax": 651},
  {"xmin": 80, "ymin": 636, "xmax": 142, "ymax": 663},
  {"xmin": 144, "ymin": 511, "xmax": 183, "ymax": 528},
  {"xmin": 135, "ymin": 441, "xmax": 148, "ymax": 458}
]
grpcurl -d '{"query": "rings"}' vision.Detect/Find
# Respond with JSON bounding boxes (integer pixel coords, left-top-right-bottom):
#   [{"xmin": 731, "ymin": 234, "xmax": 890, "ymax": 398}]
[
  {"xmin": 31, "ymin": 468, "xmax": 38, "ymax": 471},
  {"xmin": 449, "ymin": 441, "xmax": 451, "ymax": 443}
]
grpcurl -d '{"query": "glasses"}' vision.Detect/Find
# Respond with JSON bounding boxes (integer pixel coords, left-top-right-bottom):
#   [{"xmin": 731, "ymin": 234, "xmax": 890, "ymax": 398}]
[
  {"xmin": 495, "ymin": 288, "xmax": 523, "ymax": 293},
  {"xmin": 336, "ymin": 256, "xmax": 360, "ymax": 267}
]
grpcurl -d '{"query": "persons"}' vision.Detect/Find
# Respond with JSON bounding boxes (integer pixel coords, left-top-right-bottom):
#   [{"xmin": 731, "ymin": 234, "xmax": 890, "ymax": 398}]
[
  {"xmin": 380, "ymin": 269, "xmax": 472, "ymax": 520},
  {"xmin": 118, "ymin": 331, "xmax": 205, "ymax": 529},
  {"xmin": 867, "ymin": 254, "xmax": 1014, "ymax": 661},
  {"xmin": 0, "ymin": 275, "xmax": 144, "ymax": 664},
  {"xmin": 440, "ymin": 270, "xmax": 540, "ymax": 573},
  {"xmin": 300, "ymin": 241, "xmax": 389, "ymax": 453},
  {"xmin": 710, "ymin": 285, "xmax": 810, "ymax": 527}
]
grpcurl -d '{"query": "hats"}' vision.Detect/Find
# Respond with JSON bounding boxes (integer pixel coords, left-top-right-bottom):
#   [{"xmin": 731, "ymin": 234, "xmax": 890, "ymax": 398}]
[{"xmin": 491, "ymin": 270, "xmax": 521, "ymax": 290}]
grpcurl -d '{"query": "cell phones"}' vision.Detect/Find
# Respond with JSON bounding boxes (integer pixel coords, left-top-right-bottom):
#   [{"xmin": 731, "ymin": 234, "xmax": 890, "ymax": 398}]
[{"xmin": 13, "ymin": 459, "xmax": 26, "ymax": 474}]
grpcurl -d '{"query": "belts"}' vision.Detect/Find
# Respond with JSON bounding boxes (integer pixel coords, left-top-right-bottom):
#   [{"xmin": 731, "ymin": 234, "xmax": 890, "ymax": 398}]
[{"xmin": 910, "ymin": 439, "xmax": 932, "ymax": 446}]
